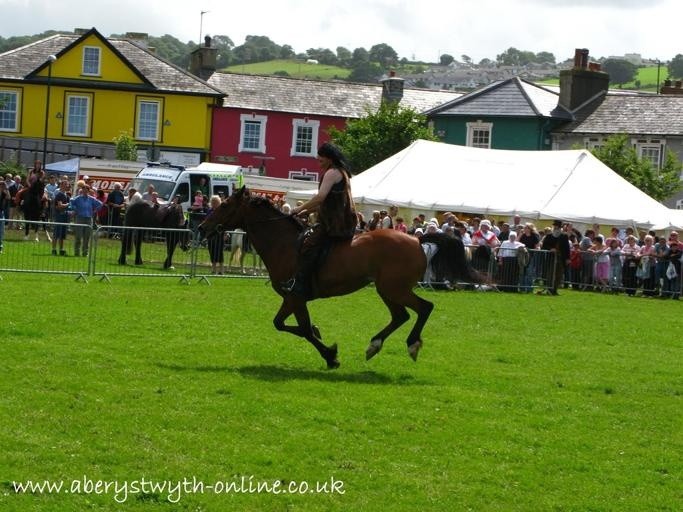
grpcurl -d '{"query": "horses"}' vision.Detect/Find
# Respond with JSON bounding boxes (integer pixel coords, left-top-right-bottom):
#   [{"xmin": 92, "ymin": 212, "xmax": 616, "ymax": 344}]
[
  {"xmin": 22, "ymin": 176, "xmax": 54, "ymax": 243},
  {"xmin": 118, "ymin": 201, "xmax": 193, "ymax": 271},
  {"xmin": 196, "ymin": 185, "xmax": 499, "ymax": 373}
]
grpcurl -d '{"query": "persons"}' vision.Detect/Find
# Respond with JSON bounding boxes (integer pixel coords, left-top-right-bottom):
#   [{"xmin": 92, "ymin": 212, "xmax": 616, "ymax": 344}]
[{"xmin": 271, "ymin": 143, "xmax": 359, "ymax": 295}]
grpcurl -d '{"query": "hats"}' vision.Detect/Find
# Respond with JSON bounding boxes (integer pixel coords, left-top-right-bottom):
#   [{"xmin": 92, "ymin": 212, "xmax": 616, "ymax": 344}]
[
  {"xmin": 83, "ymin": 175, "xmax": 90, "ymax": 180},
  {"xmin": 551, "ymin": 219, "xmax": 564, "ymax": 227}
]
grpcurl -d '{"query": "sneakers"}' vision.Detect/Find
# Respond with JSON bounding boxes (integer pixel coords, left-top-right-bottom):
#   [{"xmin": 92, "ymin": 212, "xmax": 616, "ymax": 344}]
[
  {"xmin": 52, "ymin": 248, "xmax": 175, "ymax": 270},
  {"xmin": 278, "ymin": 265, "xmax": 316, "ymax": 301},
  {"xmin": 415, "ymin": 278, "xmax": 681, "ymax": 302},
  {"xmin": 210, "ymin": 271, "xmax": 224, "ymax": 275}
]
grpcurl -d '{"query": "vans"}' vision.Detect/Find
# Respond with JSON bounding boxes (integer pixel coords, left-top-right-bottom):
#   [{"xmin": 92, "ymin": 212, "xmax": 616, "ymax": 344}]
[{"xmin": 123, "ymin": 161, "xmax": 243, "ymax": 227}]
